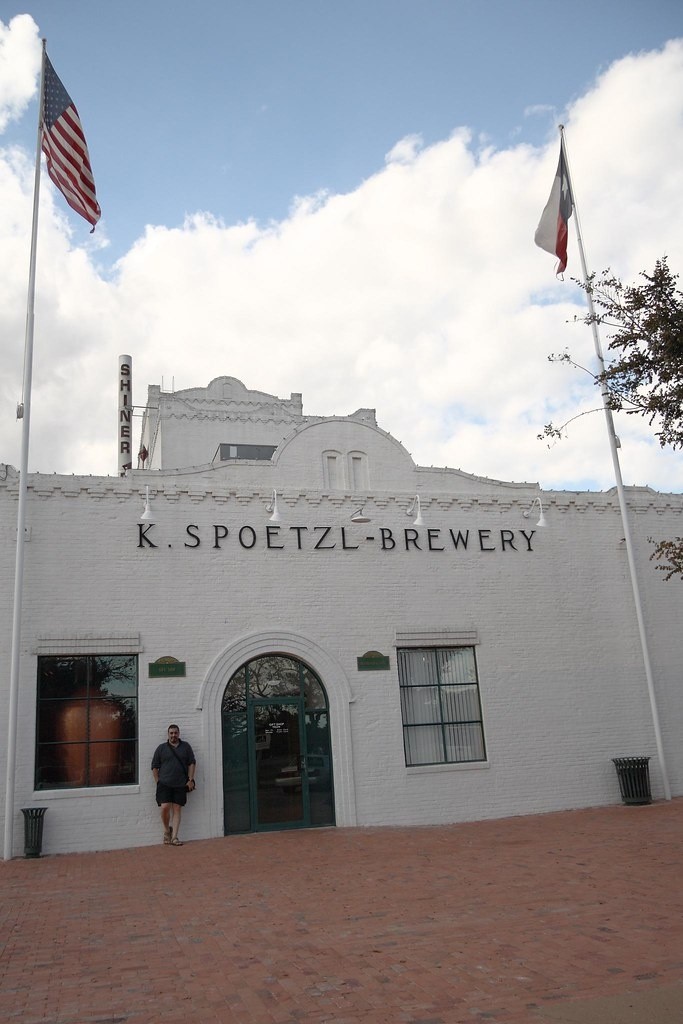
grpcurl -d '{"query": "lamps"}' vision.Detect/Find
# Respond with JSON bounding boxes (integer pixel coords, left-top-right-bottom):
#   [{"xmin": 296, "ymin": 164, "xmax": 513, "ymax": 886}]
[
  {"xmin": 351, "ymin": 507, "xmax": 372, "ymax": 523},
  {"xmin": 524, "ymin": 497, "xmax": 548, "ymax": 527},
  {"xmin": 140, "ymin": 486, "xmax": 156, "ymax": 520},
  {"xmin": 406, "ymin": 495, "xmax": 427, "ymax": 526},
  {"xmin": 139, "ymin": 444, "xmax": 149, "ymax": 468},
  {"xmin": 266, "ymin": 489, "xmax": 285, "ymax": 522}
]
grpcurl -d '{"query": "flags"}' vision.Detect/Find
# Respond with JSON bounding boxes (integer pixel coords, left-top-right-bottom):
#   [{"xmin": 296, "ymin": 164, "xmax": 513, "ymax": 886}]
[
  {"xmin": 41, "ymin": 54, "xmax": 101, "ymax": 229},
  {"xmin": 534, "ymin": 140, "xmax": 574, "ymax": 280}
]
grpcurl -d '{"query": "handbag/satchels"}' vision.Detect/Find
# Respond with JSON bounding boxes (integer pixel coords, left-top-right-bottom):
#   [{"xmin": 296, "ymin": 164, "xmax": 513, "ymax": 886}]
[{"xmin": 186, "ymin": 775, "xmax": 196, "ymax": 793}]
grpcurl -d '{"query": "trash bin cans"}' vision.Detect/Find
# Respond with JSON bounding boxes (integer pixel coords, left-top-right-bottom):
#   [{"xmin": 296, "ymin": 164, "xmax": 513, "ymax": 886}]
[
  {"xmin": 612, "ymin": 756, "xmax": 653, "ymax": 805},
  {"xmin": 21, "ymin": 808, "xmax": 47, "ymax": 858}
]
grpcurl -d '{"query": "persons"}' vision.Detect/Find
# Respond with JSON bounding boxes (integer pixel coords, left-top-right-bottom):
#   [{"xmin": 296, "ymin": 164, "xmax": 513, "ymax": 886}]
[{"xmin": 151, "ymin": 724, "xmax": 196, "ymax": 845}]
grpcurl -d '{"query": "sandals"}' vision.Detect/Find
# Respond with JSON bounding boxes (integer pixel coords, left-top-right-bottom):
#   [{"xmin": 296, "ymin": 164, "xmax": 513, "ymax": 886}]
[
  {"xmin": 171, "ymin": 837, "xmax": 184, "ymax": 845},
  {"xmin": 163, "ymin": 827, "xmax": 173, "ymax": 845}
]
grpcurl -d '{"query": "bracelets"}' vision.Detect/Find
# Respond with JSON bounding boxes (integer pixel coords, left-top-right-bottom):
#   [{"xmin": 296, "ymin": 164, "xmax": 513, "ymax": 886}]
[{"xmin": 189, "ymin": 779, "xmax": 193, "ymax": 782}]
